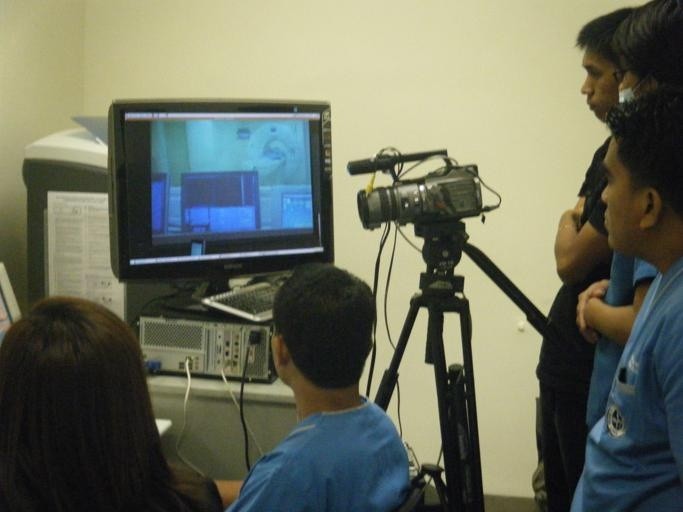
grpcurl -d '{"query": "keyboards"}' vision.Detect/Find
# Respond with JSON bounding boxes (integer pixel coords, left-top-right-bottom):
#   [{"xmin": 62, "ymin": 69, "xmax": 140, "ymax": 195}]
[{"xmin": 201, "ymin": 281, "xmax": 288, "ymax": 324}]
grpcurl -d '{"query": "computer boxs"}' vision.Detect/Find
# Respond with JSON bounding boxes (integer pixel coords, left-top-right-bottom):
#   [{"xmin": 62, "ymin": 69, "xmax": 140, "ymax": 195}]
[{"xmin": 124, "ymin": 310, "xmax": 280, "ymax": 384}]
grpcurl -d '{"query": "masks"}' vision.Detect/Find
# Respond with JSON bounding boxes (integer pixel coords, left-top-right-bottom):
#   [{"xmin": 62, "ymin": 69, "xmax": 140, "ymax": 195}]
[{"xmin": 612, "ymin": 73, "xmax": 649, "ymax": 104}]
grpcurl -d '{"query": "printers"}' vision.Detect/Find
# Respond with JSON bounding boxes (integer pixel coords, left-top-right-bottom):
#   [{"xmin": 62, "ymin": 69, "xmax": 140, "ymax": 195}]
[{"xmin": 22, "ymin": 125, "xmax": 124, "ymax": 325}]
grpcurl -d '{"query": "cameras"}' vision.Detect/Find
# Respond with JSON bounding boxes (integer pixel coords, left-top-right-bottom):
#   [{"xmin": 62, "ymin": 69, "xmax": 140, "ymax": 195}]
[{"xmin": 347, "ymin": 147, "xmax": 502, "ymax": 230}]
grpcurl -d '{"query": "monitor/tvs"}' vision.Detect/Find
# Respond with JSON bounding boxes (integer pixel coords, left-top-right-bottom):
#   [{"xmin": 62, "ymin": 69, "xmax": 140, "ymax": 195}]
[{"xmin": 107, "ymin": 97, "xmax": 335, "ymax": 312}]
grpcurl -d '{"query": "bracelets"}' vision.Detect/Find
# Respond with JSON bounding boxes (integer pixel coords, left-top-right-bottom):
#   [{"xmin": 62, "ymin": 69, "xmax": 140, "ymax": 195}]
[{"xmin": 555, "ymin": 222, "xmax": 579, "ymax": 231}]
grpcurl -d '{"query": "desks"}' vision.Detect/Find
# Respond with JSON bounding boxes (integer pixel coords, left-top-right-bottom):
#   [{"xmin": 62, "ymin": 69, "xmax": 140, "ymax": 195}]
[{"xmin": 143, "ymin": 370, "xmax": 297, "ymax": 480}]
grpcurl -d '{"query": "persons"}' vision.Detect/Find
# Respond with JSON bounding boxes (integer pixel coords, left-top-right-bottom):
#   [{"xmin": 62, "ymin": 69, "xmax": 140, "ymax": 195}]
[
  {"xmin": 533, "ymin": 8, "xmax": 642, "ymax": 511},
  {"xmin": 214, "ymin": 262, "xmax": 414, "ymax": 510},
  {"xmin": 582, "ymin": 0, "xmax": 681, "ymax": 432},
  {"xmin": 569, "ymin": 88, "xmax": 683, "ymax": 509},
  {"xmin": 0, "ymin": 296, "xmax": 226, "ymax": 512}
]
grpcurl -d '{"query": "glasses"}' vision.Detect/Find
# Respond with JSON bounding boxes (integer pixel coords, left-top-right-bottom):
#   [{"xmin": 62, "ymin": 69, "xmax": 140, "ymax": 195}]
[{"xmin": 612, "ymin": 65, "xmax": 631, "ymax": 83}]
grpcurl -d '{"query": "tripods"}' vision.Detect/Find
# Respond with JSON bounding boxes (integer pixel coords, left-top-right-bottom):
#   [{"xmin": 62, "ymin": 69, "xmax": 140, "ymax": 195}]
[{"xmin": 374, "ymin": 273, "xmax": 486, "ymax": 512}]
